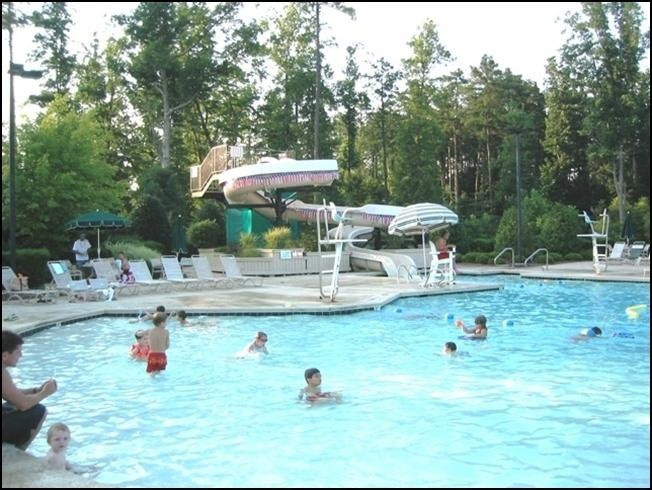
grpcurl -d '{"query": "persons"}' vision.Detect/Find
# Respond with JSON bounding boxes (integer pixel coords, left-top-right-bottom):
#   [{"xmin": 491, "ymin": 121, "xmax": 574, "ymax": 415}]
[
  {"xmin": 573, "ymin": 326, "xmax": 618, "ymax": 340},
  {"xmin": 177, "ymin": 310, "xmax": 190, "ymax": 326},
  {"xmin": 242, "ymin": 330, "xmax": 269, "ymax": 354},
  {"xmin": 136, "ymin": 312, "xmax": 171, "ymax": 372},
  {"xmin": 111, "ymin": 251, "xmax": 131, "ymax": 283},
  {"xmin": 459, "ymin": 314, "xmax": 489, "ymax": 340},
  {"xmin": 127, "ymin": 310, "xmax": 155, "ymax": 325},
  {"xmin": 298, "ymin": 367, "xmax": 339, "ymax": 405},
  {"xmin": 129, "ymin": 328, "xmax": 150, "ymax": 360},
  {"xmin": 2, "ymin": 330, "xmax": 58, "ymax": 451},
  {"xmin": 153, "ymin": 305, "xmax": 166, "ymax": 317},
  {"xmin": 69, "ymin": 230, "xmax": 93, "ymax": 286},
  {"xmin": 434, "ymin": 230, "xmax": 462, "ymax": 275},
  {"xmin": 43, "ymin": 422, "xmax": 99, "ymax": 474},
  {"xmin": 441, "ymin": 342, "xmax": 458, "ymax": 354}
]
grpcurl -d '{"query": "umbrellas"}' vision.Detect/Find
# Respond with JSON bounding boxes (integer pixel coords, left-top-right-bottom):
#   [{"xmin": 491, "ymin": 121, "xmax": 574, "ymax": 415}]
[
  {"xmin": 618, "ymin": 211, "xmax": 638, "ymax": 244},
  {"xmin": 388, "ymin": 202, "xmax": 460, "ymax": 280},
  {"xmin": 65, "ymin": 208, "xmax": 133, "ymax": 255}
]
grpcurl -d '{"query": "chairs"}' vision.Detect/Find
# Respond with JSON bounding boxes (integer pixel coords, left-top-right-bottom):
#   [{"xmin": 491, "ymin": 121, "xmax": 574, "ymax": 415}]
[
  {"xmin": 608, "ymin": 240, "xmax": 650, "ymax": 266},
  {"xmin": 428, "ymin": 240, "xmax": 454, "ymax": 284},
  {"xmin": 2, "ymin": 252, "xmax": 266, "ymax": 307}
]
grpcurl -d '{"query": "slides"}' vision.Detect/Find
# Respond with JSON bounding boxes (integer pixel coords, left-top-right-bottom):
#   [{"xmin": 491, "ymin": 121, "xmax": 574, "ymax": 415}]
[{"xmin": 219, "ymin": 159, "xmax": 418, "ymax": 278}]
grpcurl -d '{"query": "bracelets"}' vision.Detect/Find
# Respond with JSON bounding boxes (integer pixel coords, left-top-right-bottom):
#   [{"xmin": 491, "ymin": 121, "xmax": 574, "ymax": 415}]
[{"xmin": 33, "ymin": 388, "xmax": 37, "ymax": 394}]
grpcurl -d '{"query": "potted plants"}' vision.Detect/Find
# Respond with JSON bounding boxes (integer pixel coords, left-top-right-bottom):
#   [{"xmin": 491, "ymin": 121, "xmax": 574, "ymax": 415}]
[{"xmin": 185, "ymin": 219, "xmax": 351, "ymax": 273}]
